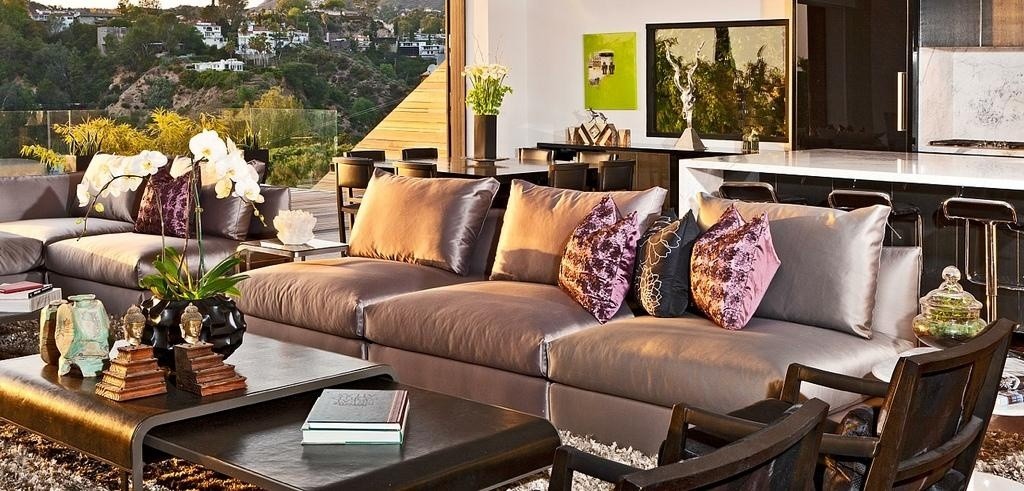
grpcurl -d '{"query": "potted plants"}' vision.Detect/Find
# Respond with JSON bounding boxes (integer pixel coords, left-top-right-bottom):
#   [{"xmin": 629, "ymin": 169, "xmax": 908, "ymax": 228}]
[{"xmin": 65, "ymin": 123, "xmax": 110, "ymax": 174}]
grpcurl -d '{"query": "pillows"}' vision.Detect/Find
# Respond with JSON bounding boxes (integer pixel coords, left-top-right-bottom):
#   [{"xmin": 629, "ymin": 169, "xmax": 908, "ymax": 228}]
[
  {"xmin": 487, "ymin": 164, "xmax": 893, "ymax": 344},
  {"xmin": 344, "ymin": 165, "xmax": 501, "ymax": 277},
  {"xmin": 73, "ymin": 152, "xmax": 271, "ymax": 239}
]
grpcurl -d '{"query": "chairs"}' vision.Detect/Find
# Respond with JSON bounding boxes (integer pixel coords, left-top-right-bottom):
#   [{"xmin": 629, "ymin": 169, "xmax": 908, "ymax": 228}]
[
  {"xmin": 936, "ymin": 192, "xmax": 1018, "ymax": 326},
  {"xmin": 331, "ymin": 156, "xmax": 375, "ymax": 244},
  {"xmin": 402, "ymin": 148, "xmax": 439, "ymax": 161},
  {"xmin": 344, "ymin": 151, "xmax": 386, "ymax": 164},
  {"xmin": 824, "ymin": 188, "xmax": 926, "ymax": 249},
  {"xmin": 547, "ymin": 165, "xmax": 590, "ymax": 191},
  {"xmin": 598, "ymin": 160, "xmax": 635, "ymax": 191},
  {"xmin": 548, "ymin": 394, "xmax": 833, "ymax": 491},
  {"xmin": 393, "ymin": 164, "xmax": 439, "ymax": 179},
  {"xmin": 780, "ymin": 314, "xmax": 1020, "ymax": 490}
]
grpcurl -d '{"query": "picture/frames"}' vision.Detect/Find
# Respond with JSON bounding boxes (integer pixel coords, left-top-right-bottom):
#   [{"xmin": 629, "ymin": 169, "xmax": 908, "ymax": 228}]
[{"xmin": 645, "ymin": 17, "xmax": 792, "ymax": 144}]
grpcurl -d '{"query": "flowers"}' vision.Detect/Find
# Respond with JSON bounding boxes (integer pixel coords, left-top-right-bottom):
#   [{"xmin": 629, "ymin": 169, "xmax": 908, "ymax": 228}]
[
  {"xmin": 458, "ymin": 30, "xmax": 516, "ymax": 116},
  {"xmin": 76, "ymin": 127, "xmax": 276, "ymax": 302}
]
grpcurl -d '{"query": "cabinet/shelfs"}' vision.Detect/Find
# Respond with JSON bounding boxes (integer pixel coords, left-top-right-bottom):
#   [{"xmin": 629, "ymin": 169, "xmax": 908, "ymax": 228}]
[{"xmin": 537, "ymin": 143, "xmax": 760, "ymax": 219}]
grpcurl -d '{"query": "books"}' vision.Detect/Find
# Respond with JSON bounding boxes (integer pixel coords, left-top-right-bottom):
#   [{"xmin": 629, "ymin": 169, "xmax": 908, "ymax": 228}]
[
  {"xmin": 307, "ymin": 389, "xmax": 408, "ymax": 430},
  {"xmin": 300, "ymin": 400, "xmax": 411, "ymax": 444}
]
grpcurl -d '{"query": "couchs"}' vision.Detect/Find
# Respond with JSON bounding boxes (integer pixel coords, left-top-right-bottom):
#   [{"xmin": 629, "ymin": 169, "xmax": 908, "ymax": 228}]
[
  {"xmin": 0, "ymin": 170, "xmax": 292, "ymax": 292},
  {"xmin": 225, "ymin": 182, "xmax": 925, "ymax": 464}
]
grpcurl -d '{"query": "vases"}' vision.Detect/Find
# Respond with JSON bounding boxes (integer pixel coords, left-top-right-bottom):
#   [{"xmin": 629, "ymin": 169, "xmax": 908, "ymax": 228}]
[
  {"xmin": 130, "ymin": 291, "xmax": 247, "ymax": 372},
  {"xmin": 473, "ymin": 114, "xmax": 498, "ymax": 161}
]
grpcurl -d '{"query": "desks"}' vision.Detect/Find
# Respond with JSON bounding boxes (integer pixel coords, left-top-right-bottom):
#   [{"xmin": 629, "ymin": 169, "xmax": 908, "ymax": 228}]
[
  {"xmin": 677, "ymin": 144, "xmax": 1024, "ymax": 358},
  {"xmin": 230, "ymin": 239, "xmax": 351, "ymax": 280},
  {"xmin": 372, "ymin": 161, "xmax": 598, "ymax": 209}
]
